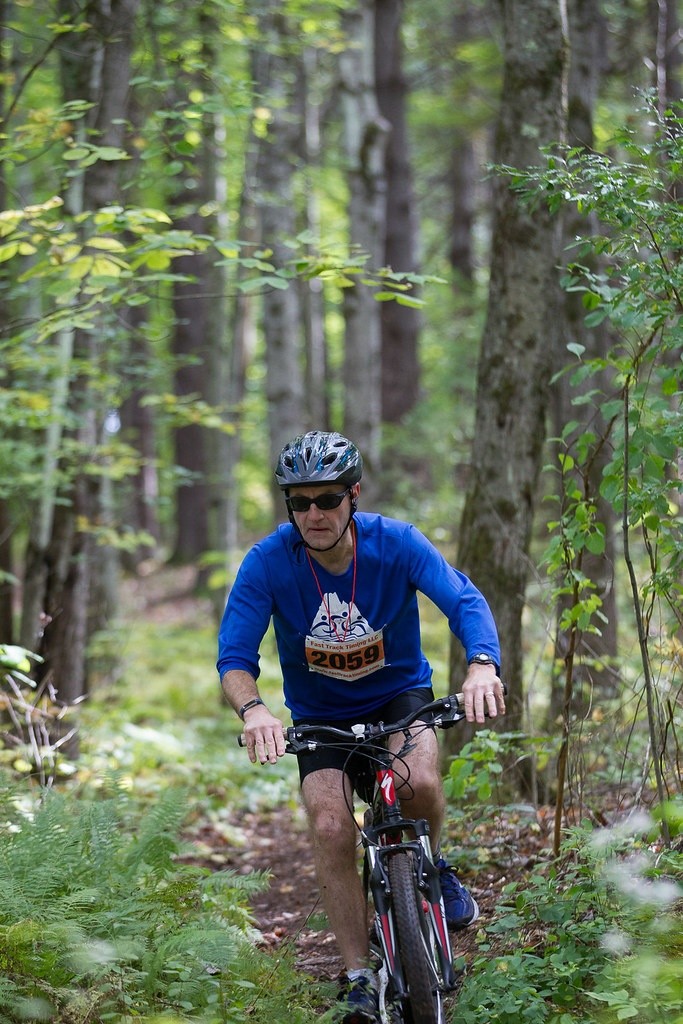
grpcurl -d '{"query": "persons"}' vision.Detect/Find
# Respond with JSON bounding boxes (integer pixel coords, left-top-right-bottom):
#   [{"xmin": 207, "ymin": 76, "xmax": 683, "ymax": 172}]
[{"xmin": 217, "ymin": 431, "xmax": 505, "ymax": 1024}]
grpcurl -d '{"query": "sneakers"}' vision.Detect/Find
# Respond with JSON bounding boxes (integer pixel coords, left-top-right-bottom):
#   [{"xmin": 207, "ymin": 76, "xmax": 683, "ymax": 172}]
[
  {"xmin": 342, "ymin": 975, "xmax": 380, "ymax": 1024},
  {"xmin": 435, "ymin": 859, "xmax": 479, "ymax": 931}
]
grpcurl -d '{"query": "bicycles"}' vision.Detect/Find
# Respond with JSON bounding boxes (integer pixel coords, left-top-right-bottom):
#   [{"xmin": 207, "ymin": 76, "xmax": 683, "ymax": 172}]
[{"xmin": 237, "ymin": 682, "xmax": 511, "ymax": 1024}]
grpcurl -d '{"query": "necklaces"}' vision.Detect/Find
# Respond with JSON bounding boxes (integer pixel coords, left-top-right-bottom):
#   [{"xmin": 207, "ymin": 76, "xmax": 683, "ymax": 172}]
[{"xmin": 306, "ymin": 520, "xmax": 356, "ymax": 642}]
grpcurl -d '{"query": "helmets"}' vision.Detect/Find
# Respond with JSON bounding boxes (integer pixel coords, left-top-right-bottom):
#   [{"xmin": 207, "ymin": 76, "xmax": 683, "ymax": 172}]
[{"xmin": 274, "ymin": 430, "xmax": 363, "ymax": 491}]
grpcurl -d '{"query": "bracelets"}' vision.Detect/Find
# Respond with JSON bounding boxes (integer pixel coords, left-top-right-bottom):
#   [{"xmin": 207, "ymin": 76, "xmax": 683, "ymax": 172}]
[{"xmin": 240, "ymin": 698, "xmax": 264, "ymax": 721}]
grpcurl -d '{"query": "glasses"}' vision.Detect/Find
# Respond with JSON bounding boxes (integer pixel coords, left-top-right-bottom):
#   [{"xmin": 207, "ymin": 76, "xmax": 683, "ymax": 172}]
[{"xmin": 285, "ymin": 489, "xmax": 350, "ymax": 512}]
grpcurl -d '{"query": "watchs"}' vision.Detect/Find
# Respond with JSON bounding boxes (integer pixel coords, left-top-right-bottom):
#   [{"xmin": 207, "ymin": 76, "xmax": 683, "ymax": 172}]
[{"xmin": 469, "ymin": 652, "xmax": 494, "ymax": 665}]
